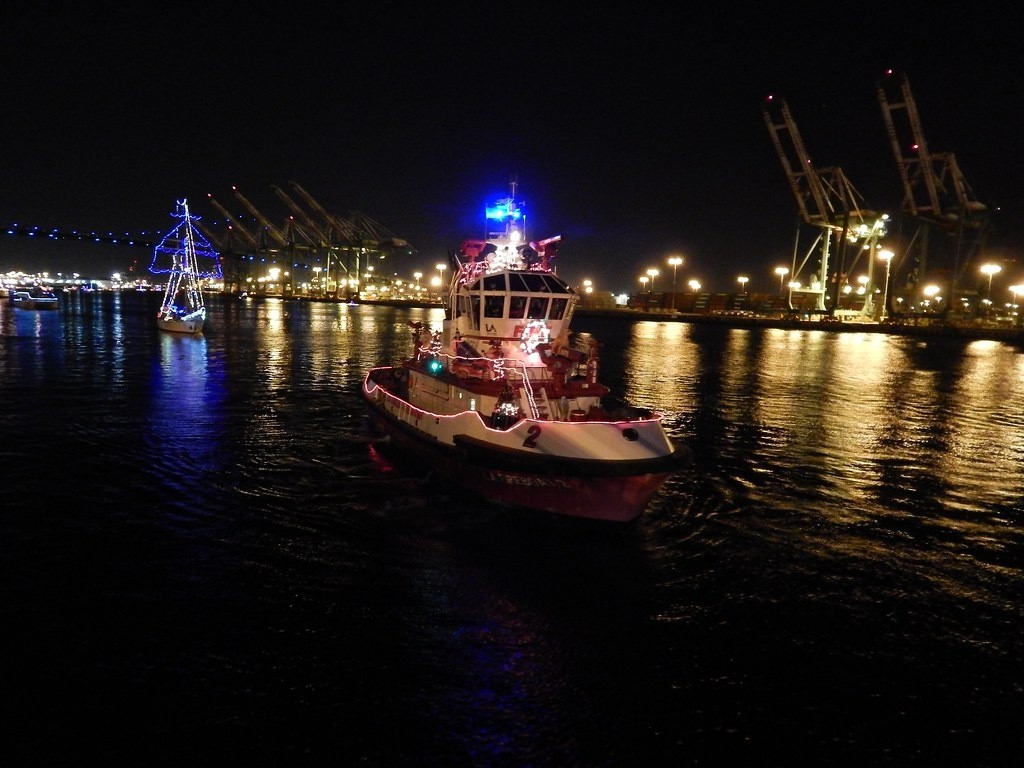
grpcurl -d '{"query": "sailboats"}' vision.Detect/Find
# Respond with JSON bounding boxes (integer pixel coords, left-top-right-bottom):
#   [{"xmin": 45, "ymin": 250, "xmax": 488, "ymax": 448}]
[{"xmin": 149, "ymin": 198, "xmax": 222, "ymax": 335}]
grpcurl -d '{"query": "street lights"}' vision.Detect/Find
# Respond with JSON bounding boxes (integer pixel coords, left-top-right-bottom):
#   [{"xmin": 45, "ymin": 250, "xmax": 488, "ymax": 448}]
[
  {"xmin": 924, "ymin": 286, "xmax": 939, "ymax": 309},
  {"xmin": 773, "ymin": 266, "xmax": 790, "ymax": 298},
  {"xmin": 436, "ymin": 263, "xmax": 447, "ymax": 283},
  {"xmin": 646, "ymin": 268, "xmax": 660, "ymax": 294},
  {"xmin": 667, "ymin": 256, "xmax": 683, "ymax": 309},
  {"xmin": 982, "ymin": 263, "xmax": 1000, "ymax": 307}
]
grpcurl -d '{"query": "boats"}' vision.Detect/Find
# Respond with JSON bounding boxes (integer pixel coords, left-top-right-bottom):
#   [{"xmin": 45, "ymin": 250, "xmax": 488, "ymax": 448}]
[
  {"xmin": 12, "ymin": 273, "xmax": 59, "ymax": 309},
  {"xmin": 360, "ymin": 178, "xmax": 684, "ymax": 525}
]
